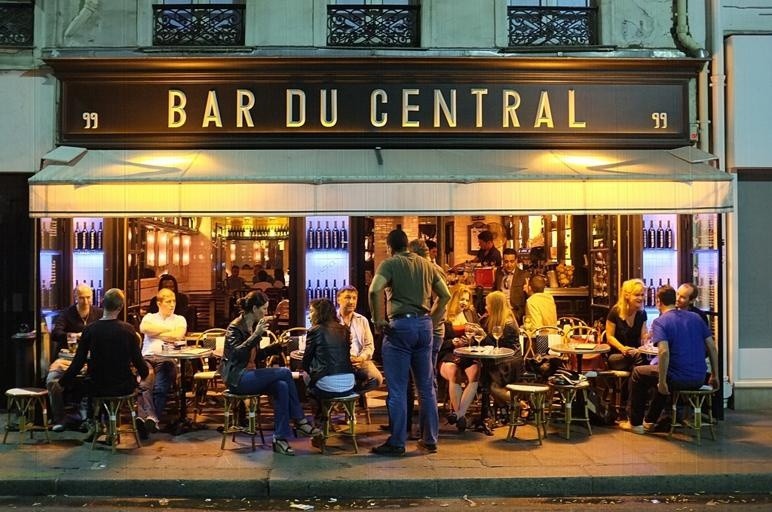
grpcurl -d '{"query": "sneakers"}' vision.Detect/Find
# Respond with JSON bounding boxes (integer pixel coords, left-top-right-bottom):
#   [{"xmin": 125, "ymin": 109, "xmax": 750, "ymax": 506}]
[
  {"xmin": 371, "ymin": 437, "xmax": 406, "ymax": 457},
  {"xmin": 445, "ymin": 413, "xmax": 467, "ymax": 432},
  {"xmin": 145, "ymin": 416, "xmax": 160, "ymax": 434},
  {"xmin": 417, "ymin": 439, "xmax": 438, "ymax": 453},
  {"xmin": 80, "ymin": 422, "xmax": 105, "ymax": 443},
  {"xmin": 135, "ymin": 417, "xmax": 149, "ymax": 440},
  {"xmin": 52, "ymin": 422, "xmax": 66, "ymax": 431},
  {"xmin": 617, "ymin": 417, "xmax": 655, "ymax": 435}
]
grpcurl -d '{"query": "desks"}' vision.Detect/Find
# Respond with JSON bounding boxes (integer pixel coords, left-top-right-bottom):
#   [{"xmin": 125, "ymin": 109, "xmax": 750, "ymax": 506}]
[
  {"xmin": 59, "ymin": 348, "xmax": 92, "ymax": 427},
  {"xmin": 550, "ymin": 343, "xmax": 611, "ymax": 427},
  {"xmin": 151, "ymin": 348, "xmax": 214, "ymax": 433},
  {"xmin": 452, "ymin": 344, "xmax": 515, "ymax": 435},
  {"xmin": 637, "ymin": 343, "xmax": 689, "ymax": 428}
]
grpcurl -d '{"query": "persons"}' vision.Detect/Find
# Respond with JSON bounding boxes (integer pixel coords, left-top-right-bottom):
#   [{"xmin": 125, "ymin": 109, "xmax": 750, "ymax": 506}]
[
  {"xmin": 222, "ymin": 261, "xmax": 287, "ymax": 291},
  {"xmin": 366, "ymin": 227, "xmax": 455, "ymax": 456},
  {"xmin": 221, "ymin": 290, "xmax": 323, "ymax": 456},
  {"xmin": 379, "ymin": 237, "xmax": 447, "ymax": 441},
  {"xmin": 47, "ymin": 287, "xmax": 154, "ymax": 442},
  {"xmin": 332, "ymin": 283, "xmax": 382, "ymax": 408},
  {"xmin": 299, "ymin": 299, "xmax": 359, "ymax": 450},
  {"xmin": 150, "ymin": 274, "xmax": 190, "ymax": 318},
  {"xmin": 48, "ymin": 285, "xmax": 106, "ymax": 433},
  {"xmin": 136, "ymin": 288, "xmax": 187, "ymax": 434}
]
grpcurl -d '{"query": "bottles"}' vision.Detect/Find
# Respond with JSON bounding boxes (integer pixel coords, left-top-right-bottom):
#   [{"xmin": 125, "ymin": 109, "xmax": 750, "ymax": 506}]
[
  {"xmin": 641, "ymin": 277, "xmax": 673, "ymax": 308},
  {"xmin": 641, "ymin": 219, "xmax": 672, "ymax": 250},
  {"xmin": 563, "ymin": 319, "xmax": 571, "ymax": 339},
  {"xmin": 162, "ymin": 337, "xmax": 187, "ymax": 351},
  {"xmin": 74, "ymin": 279, "xmax": 104, "ymax": 308},
  {"xmin": 306, "ymin": 277, "xmax": 346, "ymax": 309},
  {"xmin": 305, "ymin": 220, "xmax": 347, "ymax": 250},
  {"xmin": 583, "ymin": 219, "xmax": 609, "ymax": 300},
  {"xmin": 225, "ymin": 225, "xmax": 289, "ymax": 238},
  {"xmin": 446, "ymin": 260, "xmax": 474, "ymax": 286},
  {"xmin": 593, "ymin": 322, "xmax": 603, "ymax": 345},
  {"xmin": 72, "ymin": 221, "xmax": 105, "ymax": 252}
]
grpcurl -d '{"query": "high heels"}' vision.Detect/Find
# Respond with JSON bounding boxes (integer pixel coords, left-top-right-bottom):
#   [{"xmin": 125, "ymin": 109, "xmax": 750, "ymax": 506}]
[
  {"xmin": 293, "ymin": 422, "xmax": 321, "ymax": 438},
  {"xmin": 272, "ymin": 437, "xmax": 296, "ymax": 456}
]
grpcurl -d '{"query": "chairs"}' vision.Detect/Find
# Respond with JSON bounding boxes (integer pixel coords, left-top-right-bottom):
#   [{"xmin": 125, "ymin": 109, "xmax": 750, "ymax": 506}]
[
  {"xmin": 230, "ymin": 285, "xmax": 290, "ymax": 315},
  {"xmin": 439, "ymin": 316, "xmax": 627, "ymax": 423},
  {"xmin": 187, "ymin": 327, "xmax": 370, "ymax": 426}
]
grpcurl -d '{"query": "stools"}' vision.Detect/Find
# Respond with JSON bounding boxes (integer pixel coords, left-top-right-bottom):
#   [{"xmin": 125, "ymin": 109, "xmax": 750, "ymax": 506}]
[
  {"xmin": 504, "ymin": 383, "xmax": 550, "ymax": 445},
  {"xmin": 320, "ymin": 392, "xmax": 360, "ymax": 453},
  {"xmin": 92, "ymin": 390, "xmax": 143, "ymax": 453},
  {"xmin": 547, "ymin": 378, "xmax": 593, "ymax": 441},
  {"xmin": 5, "ymin": 387, "xmax": 51, "ymax": 447},
  {"xmin": 669, "ymin": 384, "xmax": 719, "ymax": 444},
  {"xmin": 220, "ymin": 388, "xmax": 267, "ymax": 454}
]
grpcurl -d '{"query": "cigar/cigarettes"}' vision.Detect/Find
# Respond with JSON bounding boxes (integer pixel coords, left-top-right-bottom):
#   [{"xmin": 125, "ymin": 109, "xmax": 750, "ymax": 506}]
[{"xmin": 261, "ymin": 319, "xmax": 265, "ymax": 323}]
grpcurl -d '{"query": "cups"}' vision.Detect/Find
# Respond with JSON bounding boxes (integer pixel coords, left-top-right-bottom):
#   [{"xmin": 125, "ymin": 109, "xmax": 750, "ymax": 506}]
[
  {"xmin": 299, "ymin": 336, "xmax": 306, "ymax": 351},
  {"xmin": 640, "ymin": 338, "xmax": 653, "ymax": 350},
  {"xmin": 208, "ymin": 355, "xmax": 217, "ymax": 371},
  {"xmin": 67, "ymin": 337, "xmax": 78, "ymax": 353}
]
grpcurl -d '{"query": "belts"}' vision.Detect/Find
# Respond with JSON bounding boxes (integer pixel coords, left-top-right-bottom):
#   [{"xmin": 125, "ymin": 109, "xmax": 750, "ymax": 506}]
[{"xmin": 392, "ymin": 311, "xmax": 429, "ymax": 320}]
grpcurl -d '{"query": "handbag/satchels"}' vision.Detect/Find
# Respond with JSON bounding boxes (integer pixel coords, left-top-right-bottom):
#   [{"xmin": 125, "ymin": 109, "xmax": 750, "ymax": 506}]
[{"xmin": 550, "ymin": 369, "xmax": 586, "ymax": 385}]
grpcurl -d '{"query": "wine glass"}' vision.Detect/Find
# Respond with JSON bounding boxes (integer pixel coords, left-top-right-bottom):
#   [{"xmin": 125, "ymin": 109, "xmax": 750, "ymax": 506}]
[{"xmin": 464, "ymin": 325, "xmax": 504, "ymax": 352}]
